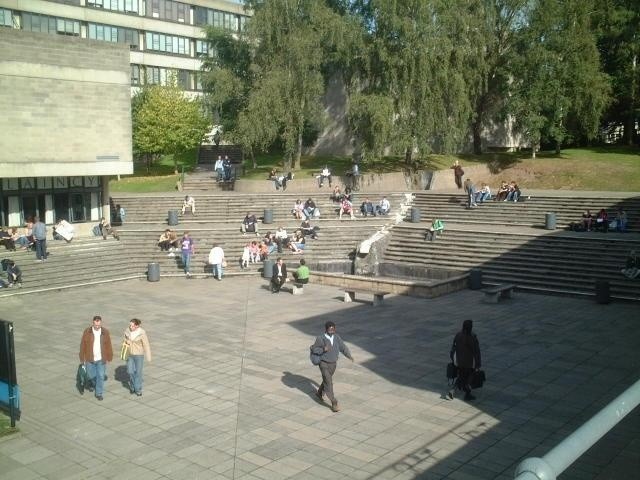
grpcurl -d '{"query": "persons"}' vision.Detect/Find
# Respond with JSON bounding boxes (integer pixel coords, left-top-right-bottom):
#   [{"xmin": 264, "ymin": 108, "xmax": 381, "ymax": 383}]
[
  {"xmin": 122, "ymin": 318, "xmax": 153, "ymax": 396},
  {"xmin": 425, "ymin": 217, "xmax": 443, "ymax": 243},
  {"xmin": 595, "ymin": 208, "xmax": 609, "ymax": 233},
  {"xmin": 114, "ymin": 204, "xmax": 125, "ymax": 223},
  {"xmin": 167, "ymin": 231, "xmax": 179, "ymax": 252},
  {"xmin": 213, "ymin": 131, "xmax": 221, "ymax": 147},
  {"xmin": 0, "ymin": 214, "xmax": 48, "ymax": 288},
  {"xmin": 214, "ymin": 155, "xmax": 224, "ymax": 182},
  {"xmin": 444, "ymin": 319, "xmax": 483, "ymax": 401},
  {"xmin": 270, "ymin": 258, "xmax": 287, "ymax": 293},
  {"xmin": 208, "ymin": 243, "xmax": 224, "ymax": 280},
  {"xmin": 310, "ymin": 322, "xmax": 355, "ymax": 413},
  {"xmin": 237, "ymin": 161, "xmax": 391, "ymax": 269},
  {"xmin": 98, "ymin": 217, "xmax": 120, "ymax": 241},
  {"xmin": 180, "ymin": 231, "xmax": 194, "ymax": 278},
  {"xmin": 614, "ymin": 207, "xmax": 629, "ymax": 232},
  {"xmin": 626, "ymin": 250, "xmax": 639, "ymax": 269},
  {"xmin": 180, "ymin": 195, "xmax": 196, "ymax": 214},
  {"xmin": 222, "ymin": 155, "xmax": 232, "ymax": 181},
  {"xmin": 582, "ymin": 209, "xmax": 593, "ymax": 232},
  {"xmin": 157, "ymin": 229, "xmax": 170, "ymax": 252},
  {"xmin": 292, "ymin": 259, "xmax": 310, "ymax": 285},
  {"xmin": 78, "ymin": 316, "xmax": 114, "ymax": 401},
  {"xmin": 446, "ymin": 160, "xmax": 522, "ymax": 209}
]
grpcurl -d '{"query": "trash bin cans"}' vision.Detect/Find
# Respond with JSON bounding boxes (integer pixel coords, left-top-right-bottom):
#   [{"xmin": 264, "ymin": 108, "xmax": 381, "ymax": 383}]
[
  {"xmin": 595, "ymin": 280, "xmax": 610, "ymax": 304},
  {"xmin": 411, "ymin": 207, "xmax": 420, "ymax": 223},
  {"xmin": 471, "ymin": 268, "xmax": 481, "ymax": 290},
  {"xmin": 148, "ymin": 263, "xmax": 160, "ymax": 282},
  {"xmin": 264, "ymin": 210, "xmax": 272, "ymax": 224},
  {"xmin": 168, "ymin": 210, "xmax": 178, "ymax": 226},
  {"xmin": 545, "ymin": 214, "xmax": 556, "ymax": 230},
  {"xmin": 263, "ymin": 259, "xmax": 275, "ymax": 277}
]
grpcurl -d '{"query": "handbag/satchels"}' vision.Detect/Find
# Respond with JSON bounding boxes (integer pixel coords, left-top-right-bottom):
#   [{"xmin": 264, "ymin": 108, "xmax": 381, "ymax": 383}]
[
  {"xmin": 472, "ymin": 370, "xmax": 486, "ymax": 388},
  {"xmin": 79, "ymin": 364, "xmax": 86, "ymax": 384},
  {"xmin": 222, "ymin": 260, "xmax": 227, "ymax": 268},
  {"xmin": 447, "ymin": 361, "xmax": 456, "ymax": 378},
  {"xmin": 121, "ymin": 344, "xmax": 130, "ymax": 361},
  {"xmin": 310, "ymin": 353, "xmax": 321, "ymax": 365}
]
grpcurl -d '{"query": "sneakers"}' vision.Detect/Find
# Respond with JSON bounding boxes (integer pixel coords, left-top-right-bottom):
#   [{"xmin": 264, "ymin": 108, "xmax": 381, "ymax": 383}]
[
  {"xmin": 332, "ymin": 404, "xmax": 339, "ymax": 412},
  {"xmin": 315, "ymin": 391, "xmax": 326, "ymax": 402}
]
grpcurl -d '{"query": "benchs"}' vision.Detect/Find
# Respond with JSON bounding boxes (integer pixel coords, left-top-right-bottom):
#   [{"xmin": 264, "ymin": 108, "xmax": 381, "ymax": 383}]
[
  {"xmin": 273, "ymin": 278, "xmax": 309, "ymax": 297},
  {"xmin": 341, "ymin": 287, "xmax": 390, "ymax": 308},
  {"xmin": 483, "ymin": 283, "xmax": 518, "ymax": 306}
]
